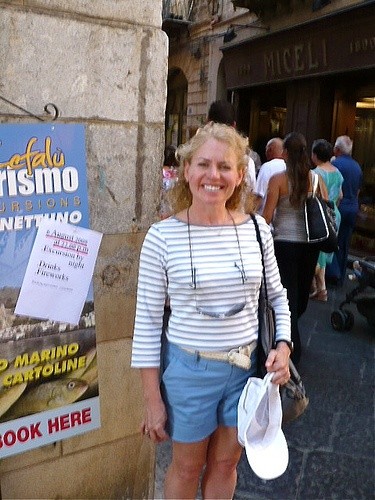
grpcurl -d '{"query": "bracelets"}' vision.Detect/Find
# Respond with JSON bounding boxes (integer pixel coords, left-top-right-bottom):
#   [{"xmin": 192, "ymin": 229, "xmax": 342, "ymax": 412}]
[{"xmin": 276, "ymin": 339, "xmax": 295, "ymax": 352}]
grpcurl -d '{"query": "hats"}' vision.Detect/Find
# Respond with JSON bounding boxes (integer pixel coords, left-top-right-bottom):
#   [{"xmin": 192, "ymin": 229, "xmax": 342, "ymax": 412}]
[{"xmin": 238, "ymin": 371, "xmax": 289, "ymax": 480}]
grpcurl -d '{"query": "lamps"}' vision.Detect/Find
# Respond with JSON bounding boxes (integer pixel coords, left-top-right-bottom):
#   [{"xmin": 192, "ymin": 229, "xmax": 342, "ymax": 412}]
[{"xmin": 222, "ymin": 23, "xmax": 271, "ymax": 43}]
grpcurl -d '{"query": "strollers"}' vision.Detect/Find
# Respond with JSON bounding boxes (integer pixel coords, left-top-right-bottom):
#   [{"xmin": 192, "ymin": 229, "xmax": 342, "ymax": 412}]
[{"xmin": 331, "ymin": 255, "xmax": 375, "ymax": 330}]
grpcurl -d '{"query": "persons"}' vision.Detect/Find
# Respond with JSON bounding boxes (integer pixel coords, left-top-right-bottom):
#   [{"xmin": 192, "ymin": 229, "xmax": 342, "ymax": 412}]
[
  {"xmin": 262, "ymin": 132, "xmax": 329, "ymax": 364},
  {"xmin": 330, "ymin": 135, "xmax": 363, "ymax": 288},
  {"xmin": 131, "ymin": 122, "xmax": 292, "ymax": 500},
  {"xmin": 309, "ymin": 139, "xmax": 345, "ymax": 302},
  {"xmin": 158, "ymin": 143, "xmax": 187, "ymax": 219},
  {"xmin": 204, "ymin": 99, "xmax": 257, "ymax": 214},
  {"xmin": 242, "ymin": 135, "xmax": 288, "ymax": 236}
]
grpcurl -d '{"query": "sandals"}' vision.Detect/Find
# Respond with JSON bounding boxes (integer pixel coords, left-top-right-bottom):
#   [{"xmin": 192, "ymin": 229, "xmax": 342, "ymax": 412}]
[{"xmin": 309, "ymin": 288, "xmax": 328, "ymax": 302}]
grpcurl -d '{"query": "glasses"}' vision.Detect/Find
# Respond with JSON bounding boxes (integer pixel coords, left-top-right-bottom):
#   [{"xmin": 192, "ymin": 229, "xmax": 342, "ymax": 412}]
[{"xmin": 194, "ymin": 262, "xmax": 247, "ymax": 318}]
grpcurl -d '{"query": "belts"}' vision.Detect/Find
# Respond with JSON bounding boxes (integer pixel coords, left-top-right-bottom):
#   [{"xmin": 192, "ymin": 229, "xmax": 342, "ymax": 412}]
[{"xmin": 176, "ymin": 341, "xmax": 258, "ymax": 371}]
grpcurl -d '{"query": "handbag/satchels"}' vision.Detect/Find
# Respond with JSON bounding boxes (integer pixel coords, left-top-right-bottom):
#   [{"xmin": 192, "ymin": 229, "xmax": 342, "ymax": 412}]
[
  {"xmin": 303, "ymin": 170, "xmax": 339, "ymax": 254},
  {"xmin": 258, "ymin": 293, "xmax": 309, "ymax": 427}
]
